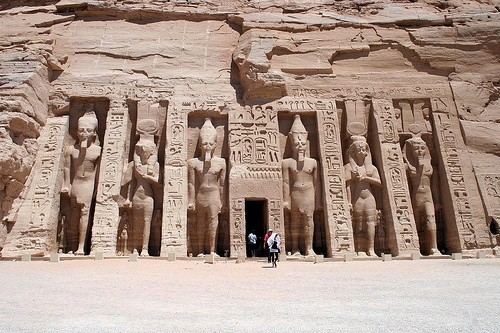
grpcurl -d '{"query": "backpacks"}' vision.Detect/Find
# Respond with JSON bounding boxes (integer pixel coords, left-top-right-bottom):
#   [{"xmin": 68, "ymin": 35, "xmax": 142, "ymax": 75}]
[{"xmin": 252, "ymin": 237, "xmax": 256, "ymax": 244}]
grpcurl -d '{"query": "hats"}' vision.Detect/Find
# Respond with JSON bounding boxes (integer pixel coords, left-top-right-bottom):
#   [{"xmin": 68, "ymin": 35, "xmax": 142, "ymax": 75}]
[{"xmin": 268, "ymin": 228, "xmax": 274, "ymax": 231}]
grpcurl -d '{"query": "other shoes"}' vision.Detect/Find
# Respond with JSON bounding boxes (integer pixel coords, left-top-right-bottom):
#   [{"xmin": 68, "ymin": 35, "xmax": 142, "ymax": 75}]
[
  {"xmin": 274, "ymin": 261, "xmax": 278, "ymax": 267},
  {"xmin": 272, "ymin": 261, "xmax": 274, "ymax": 266},
  {"xmin": 252, "ymin": 254, "xmax": 255, "ymax": 258},
  {"xmin": 268, "ymin": 258, "xmax": 271, "ymax": 262}
]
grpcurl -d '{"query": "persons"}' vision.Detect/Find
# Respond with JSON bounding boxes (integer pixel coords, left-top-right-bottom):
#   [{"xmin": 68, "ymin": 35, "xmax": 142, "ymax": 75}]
[
  {"xmin": 401, "ymin": 137, "xmax": 442, "ymax": 256},
  {"xmin": 122, "ymin": 138, "xmax": 160, "ymax": 256},
  {"xmin": 266, "ymin": 232, "xmax": 281, "ymax": 268},
  {"xmin": 343, "ymin": 135, "xmax": 382, "ymax": 259},
  {"xmin": 263, "ymin": 228, "xmax": 274, "ymax": 263},
  {"xmin": 281, "ymin": 115, "xmax": 319, "ymax": 258},
  {"xmin": 60, "ymin": 115, "xmax": 102, "ymax": 256},
  {"xmin": 186, "ymin": 117, "xmax": 226, "ymax": 258},
  {"xmin": 247, "ymin": 229, "xmax": 257, "ymax": 259}
]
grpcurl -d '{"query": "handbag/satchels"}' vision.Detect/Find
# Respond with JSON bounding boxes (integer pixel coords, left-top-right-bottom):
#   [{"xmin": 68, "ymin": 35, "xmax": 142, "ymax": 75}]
[{"xmin": 272, "ymin": 240, "xmax": 278, "ymax": 249}]
